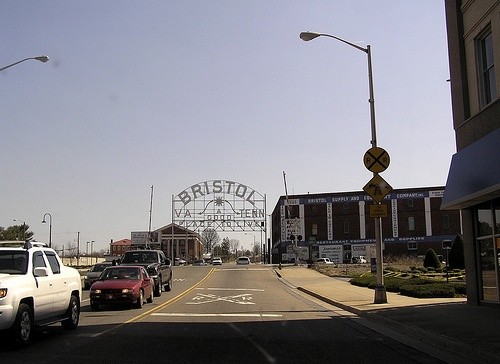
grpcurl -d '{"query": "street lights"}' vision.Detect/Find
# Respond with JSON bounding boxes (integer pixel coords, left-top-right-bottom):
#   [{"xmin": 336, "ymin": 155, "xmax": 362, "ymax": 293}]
[
  {"xmin": 299, "ymin": 31, "xmax": 394, "ymax": 305},
  {"xmin": 244, "ymin": 232, "xmax": 255, "ymax": 262},
  {"xmin": 42, "ymin": 212, "xmax": 52, "ymax": 246},
  {"xmin": 86, "ymin": 242, "xmax": 89, "ymax": 266},
  {"xmin": 91, "ymin": 240, "xmax": 95, "ymax": 265}
]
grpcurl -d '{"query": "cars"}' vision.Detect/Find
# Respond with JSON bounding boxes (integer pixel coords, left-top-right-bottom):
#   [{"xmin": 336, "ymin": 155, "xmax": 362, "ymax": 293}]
[
  {"xmin": 213, "ymin": 257, "xmax": 222, "ymax": 265},
  {"xmin": 174, "ymin": 258, "xmax": 187, "ymax": 266},
  {"xmin": 89, "ymin": 266, "xmax": 153, "ymax": 311},
  {"xmin": 84, "ymin": 262, "xmax": 120, "ymax": 291},
  {"xmin": 317, "ymin": 257, "xmax": 334, "ymax": 264},
  {"xmin": 236, "ymin": 257, "xmax": 250, "ymax": 265},
  {"xmin": 350, "ymin": 256, "xmax": 366, "ymax": 264}
]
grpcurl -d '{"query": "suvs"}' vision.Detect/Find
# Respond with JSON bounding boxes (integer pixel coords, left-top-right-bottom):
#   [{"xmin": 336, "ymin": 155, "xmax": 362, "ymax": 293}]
[
  {"xmin": 117, "ymin": 249, "xmax": 172, "ymax": 296},
  {"xmin": 0, "ymin": 239, "xmax": 82, "ymax": 344}
]
